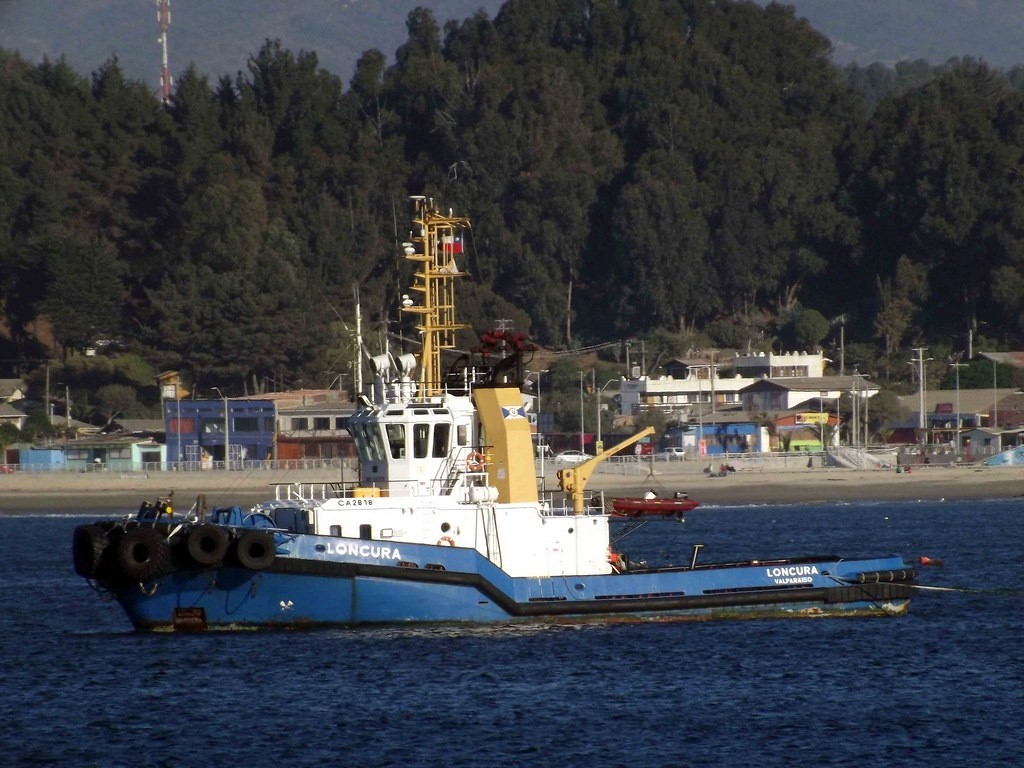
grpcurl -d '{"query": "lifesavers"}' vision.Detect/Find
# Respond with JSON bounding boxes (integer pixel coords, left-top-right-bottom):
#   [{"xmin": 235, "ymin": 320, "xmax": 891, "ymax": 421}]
[
  {"xmin": 73, "ymin": 524, "xmax": 109, "ymax": 580},
  {"xmin": 466, "ymin": 450, "xmax": 485, "ymax": 472},
  {"xmin": 235, "ymin": 529, "xmax": 277, "ymax": 569},
  {"xmin": 434, "ymin": 537, "xmax": 456, "ymax": 550},
  {"xmin": 186, "ymin": 523, "xmax": 229, "ymax": 566},
  {"xmin": 119, "ymin": 528, "xmax": 170, "ymax": 578}
]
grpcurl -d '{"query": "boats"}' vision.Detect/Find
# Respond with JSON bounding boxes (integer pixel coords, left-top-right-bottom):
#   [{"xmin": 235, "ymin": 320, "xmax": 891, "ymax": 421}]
[
  {"xmin": 71, "ymin": 195, "xmax": 924, "ymax": 637},
  {"xmin": 592, "ymin": 472, "xmax": 701, "ymax": 523}
]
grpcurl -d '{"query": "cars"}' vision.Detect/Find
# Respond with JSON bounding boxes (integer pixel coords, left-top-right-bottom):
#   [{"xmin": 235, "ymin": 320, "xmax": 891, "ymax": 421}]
[
  {"xmin": 552, "ymin": 448, "xmax": 593, "ymax": 466},
  {"xmin": 0, "ymin": 464, "xmax": 13, "ymax": 474},
  {"xmin": 655, "ymin": 446, "xmax": 686, "ymax": 461}
]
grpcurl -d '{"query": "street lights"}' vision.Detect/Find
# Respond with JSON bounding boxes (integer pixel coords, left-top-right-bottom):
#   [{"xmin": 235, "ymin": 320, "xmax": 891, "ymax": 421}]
[
  {"xmin": 596, "ymin": 379, "xmax": 620, "ymax": 454},
  {"xmin": 56, "ymin": 382, "xmax": 70, "ymax": 442},
  {"xmin": 977, "ymin": 350, "xmax": 999, "ymax": 428},
  {"xmin": 968, "ymin": 321, "xmax": 988, "ymax": 361},
  {"xmin": 39, "ymin": 363, "xmax": 50, "ymax": 417},
  {"xmin": 910, "ymin": 356, "xmax": 934, "ymax": 446},
  {"xmin": 950, "ymin": 363, "xmax": 971, "ymax": 451},
  {"xmin": 522, "ymin": 369, "xmax": 550, "ymax": 413},
  {"xmin": 818, "ymin": 356, "xmax": 835, "ymax": 451},
  {"xmin": 575, "ymin": 369, "xmax": 585, "ymax": 454},
  {"xmin": 211, "ymin": 386, "xmax": 231, "ymax": 471}
]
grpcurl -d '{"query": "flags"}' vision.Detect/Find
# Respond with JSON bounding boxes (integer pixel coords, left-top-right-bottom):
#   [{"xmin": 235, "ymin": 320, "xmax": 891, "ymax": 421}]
[{"xmin": 437, "ymin": 230, "xmax": 463, "ymax": 254}]
